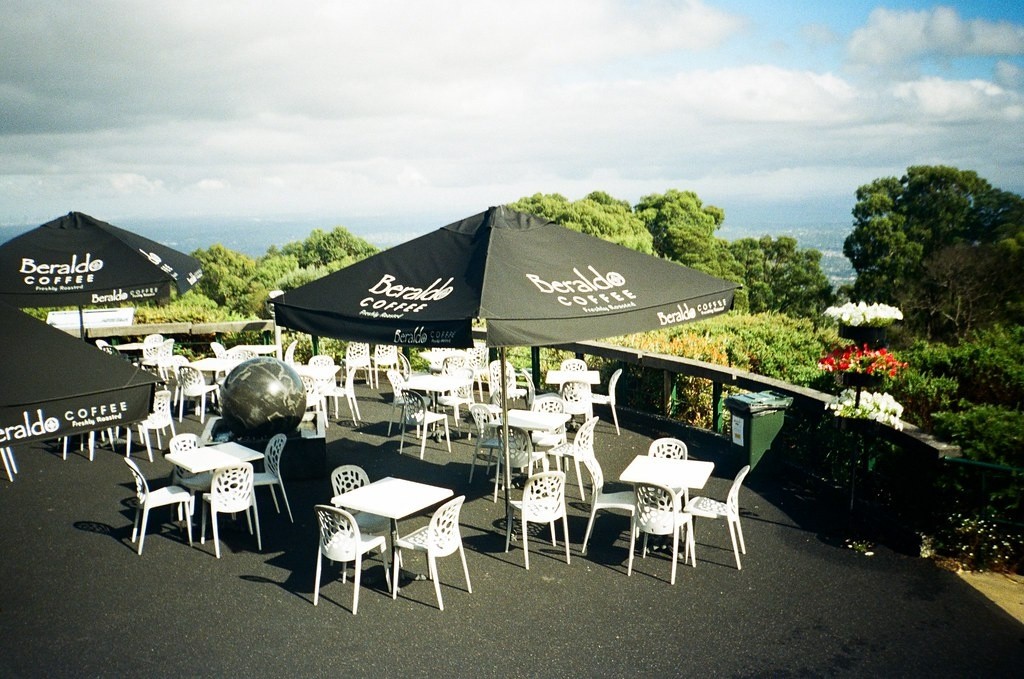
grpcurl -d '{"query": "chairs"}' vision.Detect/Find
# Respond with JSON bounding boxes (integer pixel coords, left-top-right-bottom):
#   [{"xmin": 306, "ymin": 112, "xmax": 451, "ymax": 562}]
[
  {"xmin": 628, "ymin": 480, "xmax": 696, "ymax": 586},
  {"xmin": 57, "ymin": 334, "xmax": 638, "ymax": 569},
  {"xmin": 391, "ymin": 495, "xmax": 472, "ymax": 610},
  {"xmin": 648, "ymin": 437, "xmax": 688, "ymax": 541},
  {"xmin": 313, "ymin": 505, "xmax": 392, "ymax": 615},
  {"xmin": 683, "ymin": 465, "xmax": 751, "ymax": 570}
]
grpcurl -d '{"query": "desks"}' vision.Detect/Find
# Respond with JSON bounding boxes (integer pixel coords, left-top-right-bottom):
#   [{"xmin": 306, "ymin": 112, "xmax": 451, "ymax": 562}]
[
  {"xmin": 164, "ymin": 441, "xmax": 265, "ymax": 545},
  {"xmin": 331, "ymin": 476, "xmax": 453, "ymax": 594},
  {"xmin": 545, "ymin": 369, "xmax": 601, "ymax": 433},
  {"xmin": 420, "ymin": 349, "xmax": 457, "ymax": 399},
  {"xmin": 618, "ymin": 454, "xmax": 715, "ymax": 559},
  {"xmin": 114, "ymin": 343, "xmax": 143, "ymax": 362},
  {"xmin": 227, "ymin": 344, "xmax": 281, "ymax": 354},
  {"xmin": 189, "ymin": 357, "xmax": 242, "ymax": 416},
  {"xmin": 292, "ymin": 364, "xmax": 339, "ymax": 420},
  {"xmin": 489, "ymin": 408, "xmax": 572, "ymax": 489},
  {"xmin": 406, "ymin": 374, "xmax": 474, "ymax": 441}
]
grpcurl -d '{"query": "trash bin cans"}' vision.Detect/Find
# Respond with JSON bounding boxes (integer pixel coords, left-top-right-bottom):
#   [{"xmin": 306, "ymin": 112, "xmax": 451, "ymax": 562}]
[{"xmin": 723, "ymin": 390, "xmax": 795, "ymax": 485}]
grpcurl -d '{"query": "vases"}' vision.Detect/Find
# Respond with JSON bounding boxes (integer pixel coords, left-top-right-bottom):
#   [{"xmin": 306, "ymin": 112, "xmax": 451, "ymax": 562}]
[
  {"xmin": 837, "ymin": 322, "xmax": 887, "ymax": 343},
  {"xmin": 842, "ymin": 371, "xmax": 886, "ymax": 388},
  {"xmin": 844, "ymin": 418, "xmax": 875, "ymax": 435}
]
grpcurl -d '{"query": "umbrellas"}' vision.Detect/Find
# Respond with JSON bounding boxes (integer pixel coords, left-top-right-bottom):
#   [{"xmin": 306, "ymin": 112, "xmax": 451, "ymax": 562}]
[
  {"xmin": 0, "ymin": 211, "xmax": 204, "ymax": 342},
  {"xmin": 0, "ymin": 299, "xmax": 169, "ymax": 449},
  {"xmin": 268, "ymin": 206, "xmax": 744, "ymax": 541}
]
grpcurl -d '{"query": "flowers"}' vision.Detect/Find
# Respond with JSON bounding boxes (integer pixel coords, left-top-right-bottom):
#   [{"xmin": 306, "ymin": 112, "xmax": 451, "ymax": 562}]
[
  {"xmin": 820, "ymin": 300, "xmax": 904, "ymax": 328},
  {"xmin": 824, "ymin": 389, "xmax": 904, "ymax": 432},
  {"xmin": 817, "ymin": 340, "xmax": 908, "ymax": 377}
]
